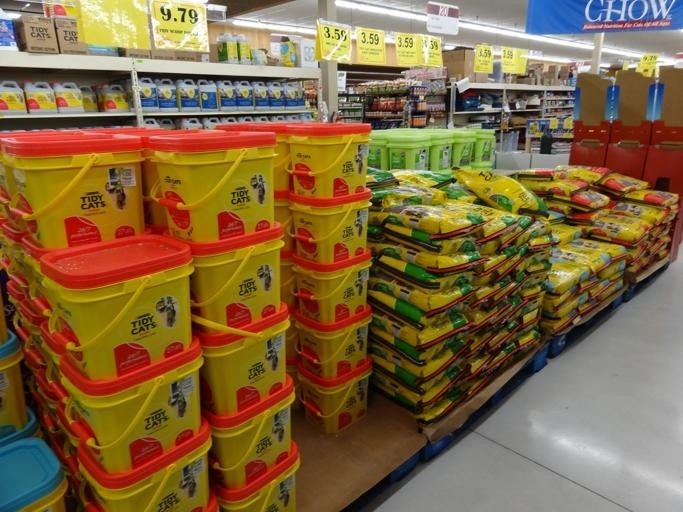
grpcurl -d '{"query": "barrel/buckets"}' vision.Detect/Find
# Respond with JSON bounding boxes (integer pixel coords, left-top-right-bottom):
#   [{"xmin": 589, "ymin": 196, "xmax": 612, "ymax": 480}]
[
  {"xmin": 0, "ymin": 6, "xmax": 376, "ymax": 512},
  {"xmin": 370, "ymin": 127, "xmax": 496, "ymax": 171}
]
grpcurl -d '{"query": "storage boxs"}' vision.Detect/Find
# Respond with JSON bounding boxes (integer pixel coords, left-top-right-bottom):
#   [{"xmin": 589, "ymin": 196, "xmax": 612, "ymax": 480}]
[
  {"xmin": 568, "ymin": 65, "xmax": 683, "ymax": 206},
  {"xmin": 14, "ymin": 15, "xmax": 487, "ymax": 83},
  {"xmin": 0, "ymin": 122, "xmax": 374, "ymax": 512}
]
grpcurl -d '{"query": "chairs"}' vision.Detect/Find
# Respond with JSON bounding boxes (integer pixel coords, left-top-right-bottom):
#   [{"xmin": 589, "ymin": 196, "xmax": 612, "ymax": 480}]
[{"xmin": 368, "ymin": 128, "xmax": 495, "ymax": 171}]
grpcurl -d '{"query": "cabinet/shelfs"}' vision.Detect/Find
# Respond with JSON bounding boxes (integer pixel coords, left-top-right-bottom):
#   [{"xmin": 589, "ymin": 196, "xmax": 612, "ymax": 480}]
[{"xmin": 0, "ymin": 53, "xmax": 576, "ymax": 139}]
